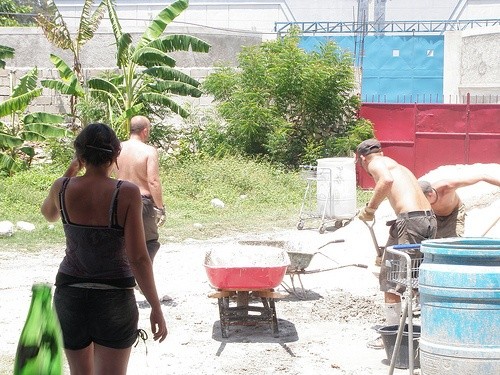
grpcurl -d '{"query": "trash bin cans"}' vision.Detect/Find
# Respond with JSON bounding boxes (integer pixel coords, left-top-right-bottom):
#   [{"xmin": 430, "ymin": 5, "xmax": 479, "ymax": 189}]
[
  {"xmin": 418, "ymin": 236, "xmax": 500, "ymax": 375},
  {"xmin": 316, "ymin": 157, "xmax": 356, "ymax": 218}
]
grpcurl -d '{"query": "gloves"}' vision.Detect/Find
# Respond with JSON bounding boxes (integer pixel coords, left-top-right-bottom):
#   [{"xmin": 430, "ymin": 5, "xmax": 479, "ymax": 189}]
[
  {"xmin": 152, "ymin": 205, "xmax": 166, "ymax": 227},
  {"xmin": 358, "ymin": 202, "xmax": 378, "ymax": 221},
  {"xmin": 375, "ymin": 256, "xmax": 382, "ymax": 266}
]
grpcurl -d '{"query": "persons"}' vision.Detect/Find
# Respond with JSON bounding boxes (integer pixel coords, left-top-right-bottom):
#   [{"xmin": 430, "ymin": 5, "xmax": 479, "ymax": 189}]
[
  {"xmin": 40, "ymin": 124, "xmax": 168, "ymax": 375},
  {"xmin": 418, "ymin": 177, "xmax": 500, "ymax": 238},
  {"xmin": 112, "ymin": 114, "xmax": 167, "ymax": 296},
  {"xmin": 356, "ymin": 137, "xmax": 438, "ymax": 350}
]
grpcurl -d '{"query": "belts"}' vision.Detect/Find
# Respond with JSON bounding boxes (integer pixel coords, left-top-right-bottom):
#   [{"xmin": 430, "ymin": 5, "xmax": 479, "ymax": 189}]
[
  {"xmin": 385, "ymin": 209, "xmax": 435, "ymax": 226},
  {"xmin": 435, "ymin": 202, "xmax": 458, "ymax": 217},
  {"xmin": 141, "ymin": 195, "xmax": 152, "ymax": 199}
]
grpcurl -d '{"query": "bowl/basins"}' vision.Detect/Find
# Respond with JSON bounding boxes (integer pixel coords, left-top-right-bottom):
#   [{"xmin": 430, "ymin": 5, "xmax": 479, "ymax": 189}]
[{"xmin": 203, "ymin": 246, "xmax": 292, "ymax": 291}]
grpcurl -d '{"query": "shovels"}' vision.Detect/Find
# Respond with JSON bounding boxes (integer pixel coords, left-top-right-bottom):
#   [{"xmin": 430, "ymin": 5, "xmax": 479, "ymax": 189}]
[{"xmin": 358, "ymin": 214, "xmax": 382, "ymax": 278}]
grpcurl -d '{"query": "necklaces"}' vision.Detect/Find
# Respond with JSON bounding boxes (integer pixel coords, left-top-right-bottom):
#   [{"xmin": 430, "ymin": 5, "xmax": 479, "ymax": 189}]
[{"xmin": 430, "ymin": 187, "xmax": 438, "ymax": 205}]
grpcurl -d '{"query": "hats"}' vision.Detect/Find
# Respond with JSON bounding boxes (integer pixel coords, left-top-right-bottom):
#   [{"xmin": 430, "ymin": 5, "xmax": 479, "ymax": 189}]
[
  {"xmin": 417, "ymin": 180, "xmax": 432, "ymax": 193},
  {"xmin": 354, "ymin": 139, "xmax": 380, "ymax": 164}
]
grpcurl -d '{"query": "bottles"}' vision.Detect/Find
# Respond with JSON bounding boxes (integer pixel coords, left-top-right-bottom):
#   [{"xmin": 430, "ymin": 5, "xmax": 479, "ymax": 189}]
[{"xmin": 14, "ymin": 284, "xmax": 64, "ymax": 375}]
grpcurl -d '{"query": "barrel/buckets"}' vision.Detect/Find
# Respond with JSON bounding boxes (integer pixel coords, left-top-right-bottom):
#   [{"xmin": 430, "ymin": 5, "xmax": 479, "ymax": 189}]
[
  {"xmin": 378, "ymin": 324, "xmax": 421, "ymax": 369},
  {"xmin": 419, "ymin": 237, "xmax": 500, "ymax": 375},
  {"xmin": 316, "ymin": 157, "xmax": 357, "ymax": 220}
]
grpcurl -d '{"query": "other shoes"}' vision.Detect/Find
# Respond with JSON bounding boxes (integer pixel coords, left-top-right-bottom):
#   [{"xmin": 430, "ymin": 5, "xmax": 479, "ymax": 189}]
[
  {"xmin": 366, "ymin": 336, "xmax": 386, "ymax": 350},
  {"xmin": 135, "ymin": 281, "xmax": 144, "ymax": 295}
]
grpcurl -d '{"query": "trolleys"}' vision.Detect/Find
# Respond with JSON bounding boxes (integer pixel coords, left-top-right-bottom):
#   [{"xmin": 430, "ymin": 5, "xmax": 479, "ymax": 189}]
[
  {"xmin": 385, "ymin": 244, "xmax": 424, "ymax": 375},
  {"xmin": 296, "ymin": 166, "xmax": 361, "ymax": 234},
  {"xmin": 203, "ymin": 244, "xmax": 292, "ymax": 338},
  {"xmin": 238, "ymin": 237, "xmax": 369, "ymax": 301}
]
